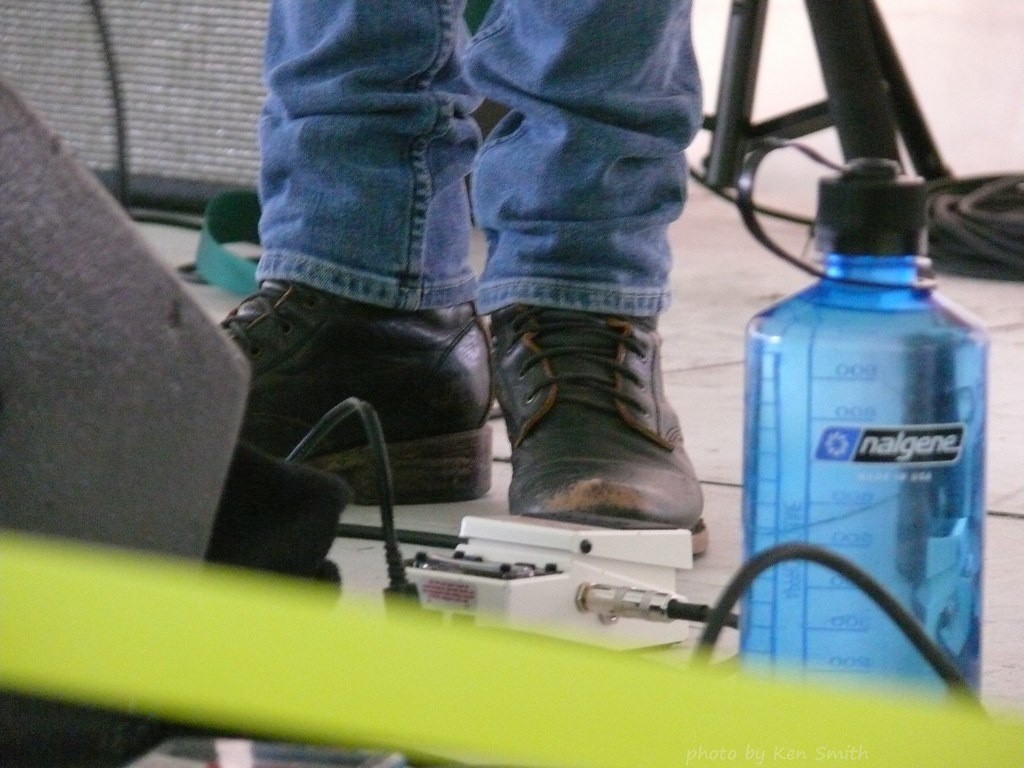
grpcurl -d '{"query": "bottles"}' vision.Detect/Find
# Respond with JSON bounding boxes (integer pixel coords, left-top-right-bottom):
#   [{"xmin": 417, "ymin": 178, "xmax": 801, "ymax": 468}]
[{"xmin": 744, "ymin": 149, "xmax": 992, "ymax": 721}]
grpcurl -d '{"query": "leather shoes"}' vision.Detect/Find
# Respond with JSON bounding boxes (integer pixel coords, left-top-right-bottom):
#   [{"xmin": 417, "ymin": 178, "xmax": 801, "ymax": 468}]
[{"xmin": 218, "ymin": 275, "xmax": 711, "ymax": 559}]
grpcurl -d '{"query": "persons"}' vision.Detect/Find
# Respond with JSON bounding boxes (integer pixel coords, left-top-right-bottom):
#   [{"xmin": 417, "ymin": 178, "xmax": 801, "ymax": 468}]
[{"xmin": 162, "ymin": 0, "xmax": 711, "ymax": 566}]
